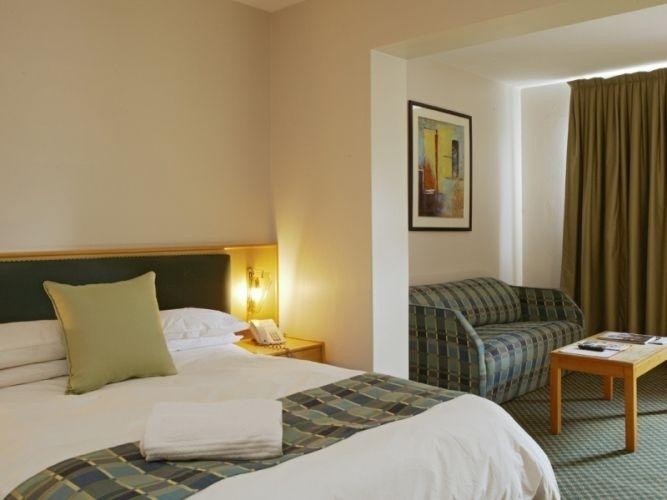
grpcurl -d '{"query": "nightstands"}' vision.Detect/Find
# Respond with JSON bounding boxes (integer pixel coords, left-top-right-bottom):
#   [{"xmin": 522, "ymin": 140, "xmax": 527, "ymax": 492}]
[{"xmin": 233, "ymin": 337, "xmax": 327, "ymax": 364}]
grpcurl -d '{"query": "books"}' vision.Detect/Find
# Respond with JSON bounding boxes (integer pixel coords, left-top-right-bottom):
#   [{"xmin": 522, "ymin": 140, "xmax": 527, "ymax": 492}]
[{"xmin": 599, "ymin": 331, "xmax": 656, "ymax": 344}]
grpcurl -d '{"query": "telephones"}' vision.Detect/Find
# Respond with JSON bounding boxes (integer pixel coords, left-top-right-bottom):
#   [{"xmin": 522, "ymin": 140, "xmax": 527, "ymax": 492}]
[{"xmin": 249, "ymin": 319, "xmax": 287, "ymax": 345}]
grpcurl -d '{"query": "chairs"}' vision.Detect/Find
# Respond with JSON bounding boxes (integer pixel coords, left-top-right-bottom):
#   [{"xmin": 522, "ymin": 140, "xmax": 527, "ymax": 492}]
[{"xmin": 0, "ymin": 270, "xmax": 248, "ymax": 395}]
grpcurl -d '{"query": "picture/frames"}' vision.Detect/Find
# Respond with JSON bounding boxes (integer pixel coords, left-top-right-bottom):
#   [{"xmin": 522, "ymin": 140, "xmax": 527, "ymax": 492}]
[{"xmin": 407, "ymin": 99, "xmax": 472, "ymax": 231}]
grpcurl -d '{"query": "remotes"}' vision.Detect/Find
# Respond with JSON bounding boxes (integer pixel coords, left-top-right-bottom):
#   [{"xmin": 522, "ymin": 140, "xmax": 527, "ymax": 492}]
[{"xmin": 578, "ymin": 343, "xmax": 605, "ymax": 351}]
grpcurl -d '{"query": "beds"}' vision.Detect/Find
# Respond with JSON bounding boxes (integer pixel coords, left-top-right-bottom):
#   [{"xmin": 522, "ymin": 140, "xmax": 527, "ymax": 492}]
[{"xmin": 0, "ymin": 254, "xmax": 513, "ymax": 500}]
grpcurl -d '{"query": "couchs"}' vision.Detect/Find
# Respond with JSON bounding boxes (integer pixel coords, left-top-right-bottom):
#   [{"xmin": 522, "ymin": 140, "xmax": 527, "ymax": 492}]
[{"xmin": 408, "ymin": 276, "xmax": 583, "ymax": 405}]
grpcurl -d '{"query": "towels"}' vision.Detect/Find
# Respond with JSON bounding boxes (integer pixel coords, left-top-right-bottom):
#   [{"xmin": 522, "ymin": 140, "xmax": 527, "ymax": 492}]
[{"xmin": 136, "ymin": 399, "xmax": 283, "ymax": 460}]
